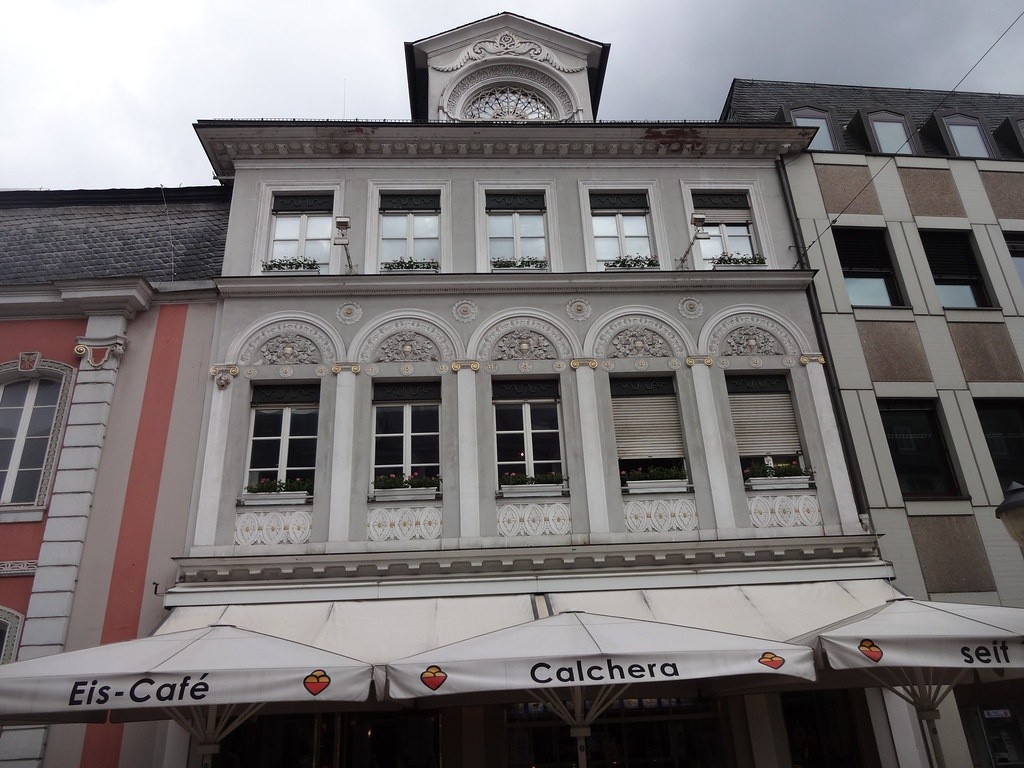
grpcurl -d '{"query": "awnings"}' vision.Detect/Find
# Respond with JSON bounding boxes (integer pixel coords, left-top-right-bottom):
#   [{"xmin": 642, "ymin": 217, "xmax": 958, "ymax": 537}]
[{"xmin": 144, "ymin": 560, "xmax": 909, "ymax": 666}]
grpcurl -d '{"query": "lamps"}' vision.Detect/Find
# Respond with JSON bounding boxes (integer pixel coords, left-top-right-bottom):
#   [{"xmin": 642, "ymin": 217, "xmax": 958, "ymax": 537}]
[
  {"xmin": 333, "ymin": 215, "xmax": 354, "ymax": 271},
  {"xmin": 673, "ymin": 211, "xmax": 711, "ymax": 270}
]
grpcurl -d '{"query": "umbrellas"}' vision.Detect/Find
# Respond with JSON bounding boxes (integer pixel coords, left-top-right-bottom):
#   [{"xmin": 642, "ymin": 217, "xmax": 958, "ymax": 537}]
[
  {"xmin": 784, "ymin": 598, "xmax": 1024, "ymax": 768},
  {"xmin": 0, "ymin": 623, "xmax": 373, "ymax": 768},
  {"xmin": 370, "ymin": 608, "xmax": 817, "ymax": 768}
]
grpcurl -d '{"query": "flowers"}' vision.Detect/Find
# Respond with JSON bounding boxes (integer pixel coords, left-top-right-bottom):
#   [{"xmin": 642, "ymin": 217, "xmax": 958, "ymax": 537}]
[
  {"xmin": 743, "ymin": 461, "xmax": 774, "ymax": 479},
  {"xmin": 260, "ymin": 254, "xmax": 767, "ymax": 272},
  {"xmin": 497, "ymin": 472, "xmax": 531, "ymax": 485},
  {"xmin": 620, "ymin": 464, "xmax": 688, "ymax": 481},
  {"xmin": 403, "ymin": 471, "xmax": 443, "ymax": 488},
  {"xmin": 371, "ymin": 473, "xmax": 410, "ymax": 489},
  {"xmin": 277, "ymin": 477, "xmax": 314, "ymax": 494},
  {"xmin": 529, "ymin": 471, "xmax": 570, "ymax": 485},
  {"xmin": 770, "ymin": 460, "xmax": 817, "ymax": 477},
  {"xmin": 242, "ymin": 476, "xmax": 283, "ymax": 493}
]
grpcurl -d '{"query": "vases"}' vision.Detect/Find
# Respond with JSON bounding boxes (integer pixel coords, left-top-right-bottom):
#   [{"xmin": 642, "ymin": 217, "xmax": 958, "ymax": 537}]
[
  {"xmin": 604, "ymin": 267, "xmax": 660, "ymax": 272},
  {"xmin": 711, "ymin": 264, "xmax": 768, "ymax": 272},
  {"xmin": 243, "ymin": 492, "xmax": 309, "ymax": 505},
  {"xmin": 491, "ymin": 268, "xmax": 548, "ymax": 273},
  {"xmin": 373, "ymin": 486, "xmax": 437, "ymax": 501},
  {"xmin": 744, "ymin": 475, "xmax": 811, "ymax": 490},
  {"xmin": 624, "ymin": 479, "xmax": 688, "ymax": 494},
  {"xmin": 500, "ymin": 484, "xmax": 564, "ymax": 498},
  {"xmin": 379, "ymin": 268, "xmax": 439, "ymax": 275},
  {"xmin": 261, "ymin": 269, "xmax": 320, "ymax": 275}
]
